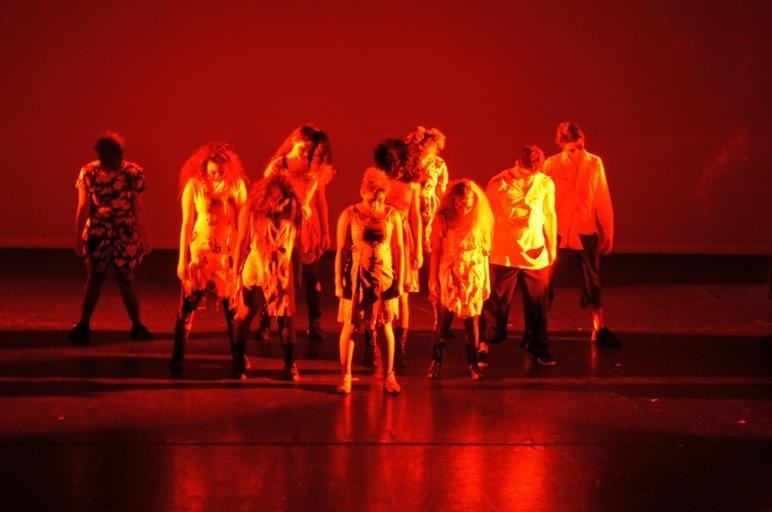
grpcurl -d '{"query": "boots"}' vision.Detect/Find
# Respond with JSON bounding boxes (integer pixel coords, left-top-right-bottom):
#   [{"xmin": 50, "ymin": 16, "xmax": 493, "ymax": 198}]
[
  {"xmin": 364, "ymin": 329, "xmax": 380, "ymax": 368},
  {"xmin": 395, "ymin": 327, "xmax": 409, "ymax": 367}
]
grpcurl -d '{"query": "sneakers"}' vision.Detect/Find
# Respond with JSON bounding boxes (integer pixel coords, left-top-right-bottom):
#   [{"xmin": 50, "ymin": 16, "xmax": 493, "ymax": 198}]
[
  {"xmin": 256, "ymin": 321, "xmax": 270, "ymax": 340},
  {"xmin": 337, "ymin": 376, "xmax": 351, "ymax": 394},
  {"xmin": 286, "ymin": 364, "xmax": 300, "ymax": 382},
  {"xmin": 591, "ymin": 326, "xmax": 621, "ymax": 348},
  {"xmin": 132, "ymin": 324, "xmax": 156, "ymax": 339},
  {"xmin": 384, "ymin": 377, "xmax": 400, "ymax": 394},
  {"xmin": 170, "ymin": 359, "xmax": 183, "ymax": 375},
  {"xmin": 536, "ymin": 353, "xmax": 555, "ymax": 366},
  {"xmin": 428, "ymin": 360, "xmax": 441, "ymax": 378},
  {"xmin": 68, "ymin": 325, "xmax": 89, "ymax": 341},
  {"xmin": 309, "ymin": 323, "xmax": 324, "ymax": 340},
  {"xmin": 480, "ymin": 342, "xmax": 489, "ymax": 358},
  {"xmin": 466, "ymin": 363, "xmax": 483, "ymax": 380},
  {"xmin": 232, "ymin": 352, "xmax": 250, "ymax": 379}
]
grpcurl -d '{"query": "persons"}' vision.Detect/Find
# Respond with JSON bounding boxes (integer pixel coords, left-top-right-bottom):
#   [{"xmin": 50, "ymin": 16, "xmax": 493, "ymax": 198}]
[
  {"xmin": 171, "ymin": 122, "xmax": 613, "ymax": 395},
  {"xmin": 67, "ymin": 135, "xmax": 152, "ymax": 343}
]
